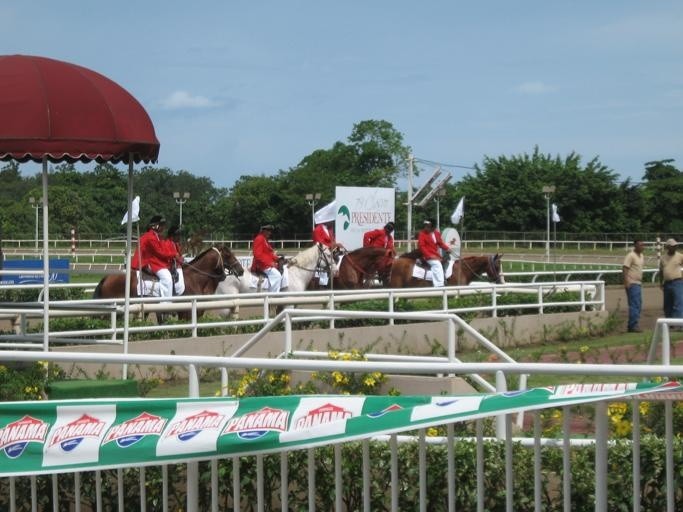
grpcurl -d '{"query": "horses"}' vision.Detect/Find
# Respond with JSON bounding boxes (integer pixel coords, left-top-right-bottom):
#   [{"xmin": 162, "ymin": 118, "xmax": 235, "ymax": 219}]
[
  {"xmin": 275, "ymin": 245, "xmax": 395, "ymax": 315},
  {"xmin": 215, "ymin": 241, "xmax": 340, "ymax": 319},
  {"xmin": 382, "ymin": 252, "xmax": 506, "ymax": 302},
  {"xmin": 90, "ymin": 244, "xmax": 244, "ymax": 323}
]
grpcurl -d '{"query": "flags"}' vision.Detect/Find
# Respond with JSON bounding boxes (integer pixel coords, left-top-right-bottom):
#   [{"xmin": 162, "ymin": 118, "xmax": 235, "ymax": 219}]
[
  {"xmin": 313, "ymin": 199, "xmax": 336, "ymax": 224},
  {"xmin": 120, "ymin": 195, "xmax": 139, "ymax": 226},
  {"xmin": 450, "ymin": 196, "xmax": 463, "ymax": 225}
]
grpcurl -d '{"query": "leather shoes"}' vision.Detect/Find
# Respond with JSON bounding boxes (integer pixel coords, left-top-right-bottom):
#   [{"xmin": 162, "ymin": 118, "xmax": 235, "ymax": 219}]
[{"xmin": 627, "ymin": 328, "xmax": 643, "ymax": 333}]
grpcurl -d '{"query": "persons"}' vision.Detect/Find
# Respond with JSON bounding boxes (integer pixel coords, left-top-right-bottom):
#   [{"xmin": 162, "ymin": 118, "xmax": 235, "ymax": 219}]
[
  {"xmin": 167, "ymin": 225, "xmax": 182, "ymax": 254},
  {"xmin": 311, "ymin": 221, "xmax": 339, "ymax": 286},
  {"xmin": 416, "ymin": 219, "xmax": 450, "ymax": 290},
  {"xmin": 658, "ymin": 238, "xmax": 683, "ymax": 333},
  {"xmin": 130, "ymin": 218, "xmax": 178, "ymax": 305},
  {"xmin": 622, "ymin": 240, "xmax": 645, "ymax": 332},
  {"xmin": 363, "ymin": 222, "xmax": 396, "ymax": 257},
  {"xmin": 157, "ymin": 220, "xmax": 185, "ymax": 295},
  {"xmin": 250, "ymin": 225, "xmax": 282, "ymax": 293}
]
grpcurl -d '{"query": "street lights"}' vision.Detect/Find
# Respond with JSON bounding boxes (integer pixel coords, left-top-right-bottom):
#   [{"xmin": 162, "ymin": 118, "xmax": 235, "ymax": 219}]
[
  {"xmin": 543, "ymin": 185, "xmax": 556, "ymax": 262},
  {"xmin": 30, "ymin": 197, "xmax": 43, "ymax": 253},
  {"xmin": 306, "ymin": 193, "xmax": 321, "ymax": 232},
  {"xmin": 433, "ymin": 189, "xmax": 446, "ymax": 232},
  {"xmin": 173, "ymin": 192, "xmax": 190, "ymax": 229}
]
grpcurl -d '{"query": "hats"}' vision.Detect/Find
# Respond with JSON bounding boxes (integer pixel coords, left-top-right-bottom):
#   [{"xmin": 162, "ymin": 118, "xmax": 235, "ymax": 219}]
[
  {"xmin": 260, "ymin": 222, "xmax": 276, "ymax": 232},
  {"xmin": 664, "ymin": 238, "xmax": 683, "ymax": 247},
  {"xmin": 386, "ymin": 222, "xmax": 395, "ymax": 230},
  {"xmin": 148, "ymin": 214, "xmax": 168, "ymax": 226},
  {"xmin": 168, "ymin": 226, "xmax": 182, "ymax": 236},
  {"xmin": 423, "ymin": 218, "xmax": 437, "ymax": 229}
]
